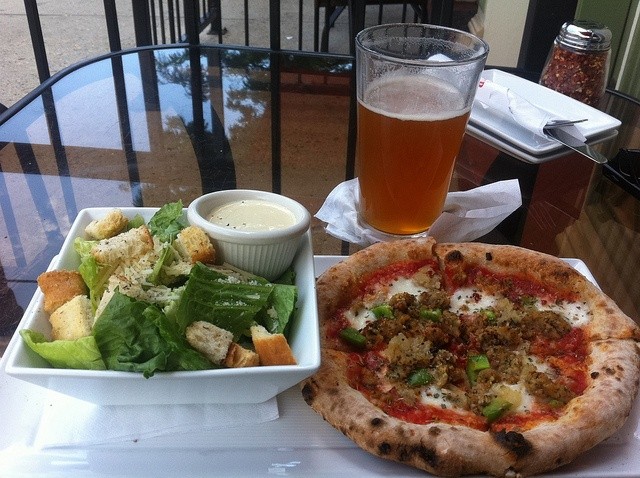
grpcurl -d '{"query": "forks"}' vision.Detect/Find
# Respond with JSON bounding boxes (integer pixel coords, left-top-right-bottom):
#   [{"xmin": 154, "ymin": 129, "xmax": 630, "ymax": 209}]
[
  {"xmin": 544, "ymin": 118, "xmax": 588, "ymax": 128},
  {"xmin": 527, "ymin": 202, "xmax": 557, "ymax": 231}
]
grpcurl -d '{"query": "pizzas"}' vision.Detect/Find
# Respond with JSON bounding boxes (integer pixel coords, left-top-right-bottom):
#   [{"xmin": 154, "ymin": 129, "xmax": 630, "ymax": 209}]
[{"xmin": 298, "ymin": 236, "xmax": 640, "ymax": 478}]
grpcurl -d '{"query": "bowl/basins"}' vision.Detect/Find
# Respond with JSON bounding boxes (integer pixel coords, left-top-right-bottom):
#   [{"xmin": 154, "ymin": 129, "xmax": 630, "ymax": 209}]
[{"xmin": 186, "ymin": 189, "xmax": 312, "ymax": 281}]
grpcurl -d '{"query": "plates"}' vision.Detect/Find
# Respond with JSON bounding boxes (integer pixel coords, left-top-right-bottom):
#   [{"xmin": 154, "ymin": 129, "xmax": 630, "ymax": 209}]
[
  {"xmin": 5, "ymin": 207, "xmax": 323, "ymax": 408},
  {"xmin": 465, "ymin": 124, "xmax": 620, "ymax": 164},
  {"xmin": 469, "ymin": 68, "xmax": 622, "ymax": 156}
]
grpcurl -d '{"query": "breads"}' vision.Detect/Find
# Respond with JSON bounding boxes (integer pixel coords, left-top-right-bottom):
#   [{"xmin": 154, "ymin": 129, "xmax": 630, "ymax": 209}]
[
  {"xmin": 92, "ymin": 225, "xmax": 154, "ymax": 271},
  {"xmin": 185, "ymin": 320, "xmax": 232, "ymax": 364},
  {"xmin": 36, "ymin": 269, "xmax": 90, "ymax": 313},
  {"xmin": 225, "ymin": 345, "xmax": 258, "ymax": 367},
  {"xmin": 174, "ymin": 225, "xmax": 217, "ymax": 264},
  {"xmin": 49, "ymin": 294, "xmax": 95, "ymax": 339},
  {"xmin": 251, "ymin": 323, "xmax": 297, "ymax": 365},
  {"xmin": 93, "ymin": 275, "xmax": 151, "ymax": 328},
  {"xmin": 85, "ymin": 211, "xmax": 128, "ymax": 240}
]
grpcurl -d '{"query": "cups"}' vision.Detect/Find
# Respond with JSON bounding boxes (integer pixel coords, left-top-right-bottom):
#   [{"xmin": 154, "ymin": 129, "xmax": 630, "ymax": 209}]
[{"xmin": 354, "ymin": 20, "xmax": 489, "ymax": 243}]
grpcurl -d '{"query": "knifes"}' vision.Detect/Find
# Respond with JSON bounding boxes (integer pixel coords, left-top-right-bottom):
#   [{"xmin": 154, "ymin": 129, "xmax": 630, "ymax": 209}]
[
  {"xmin": 543, "ymin": 201, "xmax": 588, "ymax": 224},
  {"xmin": 544, "ymin": 128, "xmax": 640, "ymax": 194}
]
